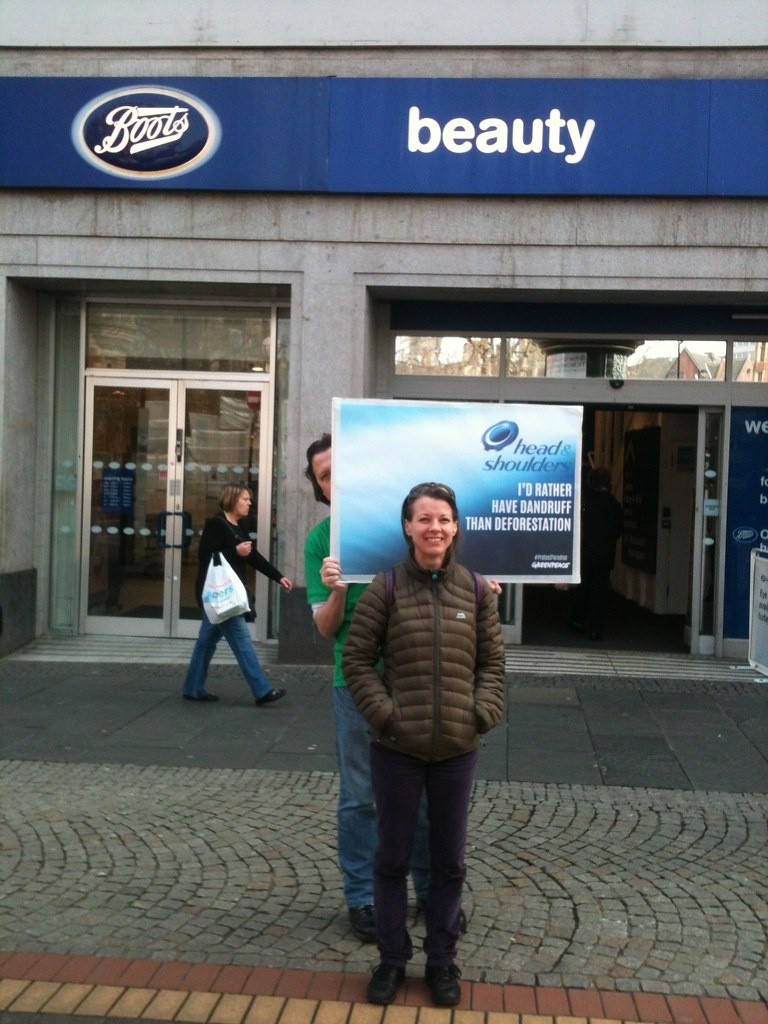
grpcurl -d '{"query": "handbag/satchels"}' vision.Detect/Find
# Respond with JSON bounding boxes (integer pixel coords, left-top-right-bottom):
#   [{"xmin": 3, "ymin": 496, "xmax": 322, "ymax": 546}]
[{"xmin": 202, "ymin": 552, "xmax": 251, "ymax": 624}]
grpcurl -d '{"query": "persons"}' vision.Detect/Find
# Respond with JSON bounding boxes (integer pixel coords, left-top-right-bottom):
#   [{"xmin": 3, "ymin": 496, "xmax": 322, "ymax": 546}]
[
  {"xmin": 571, "ymin": 467, "xmax": 622, "ymax": 640},
  {"xmin": 305, "ymin": 433, "xmax": 502, "ymax": 943},
  {"xmin": 184, "ymin": 483, "xmax": 292, "ymax": 706},
  {"xmin": 343, "ymin": 482, "xmax": 506, "ymax": 1005}
]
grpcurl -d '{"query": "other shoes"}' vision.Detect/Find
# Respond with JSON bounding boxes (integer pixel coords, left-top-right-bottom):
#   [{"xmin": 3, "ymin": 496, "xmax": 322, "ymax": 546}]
[
  {"xmin": 425, "ymin": 964, "xmax": 462, "ymax": 1005},
  {"xmin": 348, "ymin": 905, "xmax": 377, "ymax": 942},
  {"xmin": 256, "ymin": 688, "xmax": 285, "ymax": 707},
  {"xmin": 367, "ymin": 963, "xmax": 405, "ymax": 1003},
  {"xmin": 417, "ymin": 898, "xmax": 466, "ymax": 929},
  {"xmin": 183, "ymin": 694, "xmax": 218, "ymax": 701}
]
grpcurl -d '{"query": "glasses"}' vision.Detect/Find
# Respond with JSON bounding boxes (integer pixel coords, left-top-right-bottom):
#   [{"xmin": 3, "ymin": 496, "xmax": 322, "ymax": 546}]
[{"xmin": 409, "ymin": 482, "xmax": 455, "ymax": 502}]
